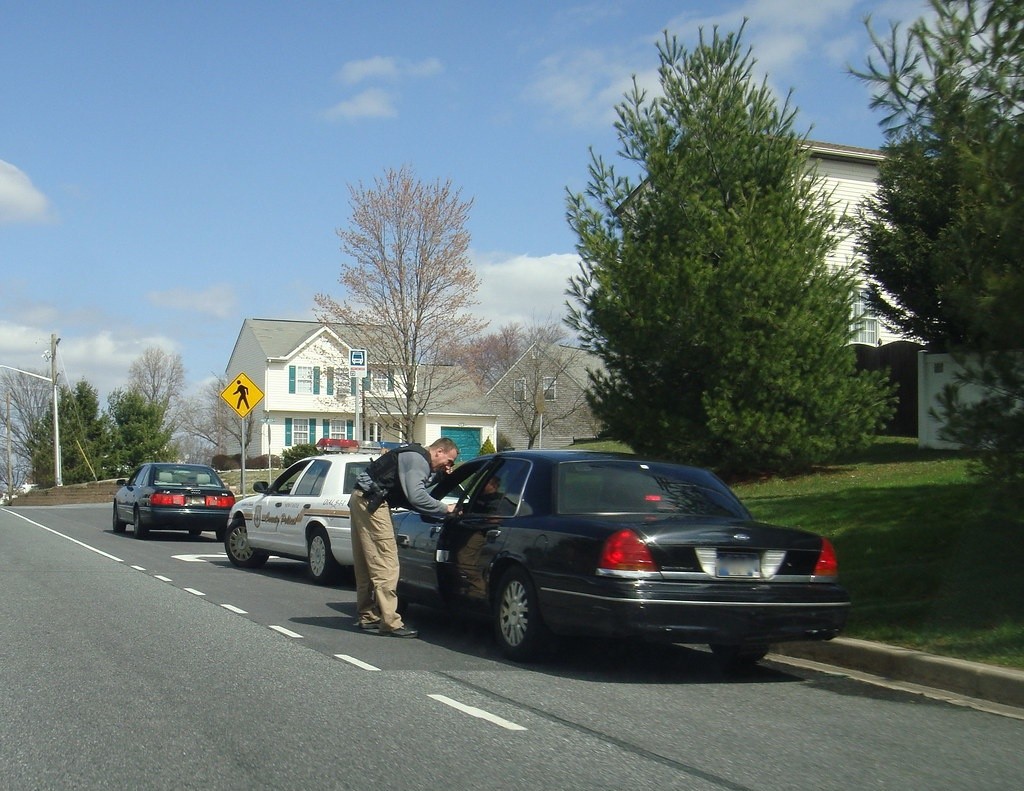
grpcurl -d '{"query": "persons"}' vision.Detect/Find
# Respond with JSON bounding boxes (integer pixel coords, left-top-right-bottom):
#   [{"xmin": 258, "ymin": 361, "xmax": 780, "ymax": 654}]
[
  {"xmin": 348, "ymin": 438, "xmax": 460, "ymax": 639},
  {"xmin": 459, "ymin": 475, "xmax": 506, "ymax": 600}
]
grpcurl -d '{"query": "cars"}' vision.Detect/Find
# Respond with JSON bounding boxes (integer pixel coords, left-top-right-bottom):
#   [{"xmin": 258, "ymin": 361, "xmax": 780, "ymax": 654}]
[
  {"xmin": 391, "ymin": 449, "xmax": 854, "ymax": 664},
  {"xmin": 223, "ymin": 438, "xmax": 471, "ymax": 586},
  {"xmin": 112, "ymin": 462, "xmax": 235, "ymax": 543}
]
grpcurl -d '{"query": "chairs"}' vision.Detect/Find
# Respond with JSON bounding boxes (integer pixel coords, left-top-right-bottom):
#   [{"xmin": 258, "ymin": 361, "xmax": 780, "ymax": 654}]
[
  {"xmin": 158, "ymin": 472, "xmax": 173, "ymax": 484},
  {"xmin": 196, "ymin": 473, "xmax": 210, "ymax": 484}
]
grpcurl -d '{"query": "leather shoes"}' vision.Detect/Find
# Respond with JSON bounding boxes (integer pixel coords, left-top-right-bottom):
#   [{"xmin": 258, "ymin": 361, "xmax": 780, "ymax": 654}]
[
  {"xmin": 379, "ymin": 626, "xmax": 417, "ymax": 637},
  {"xmin": 359, "ymin": 619, "xmax": 382, "ymax": 629}
]
